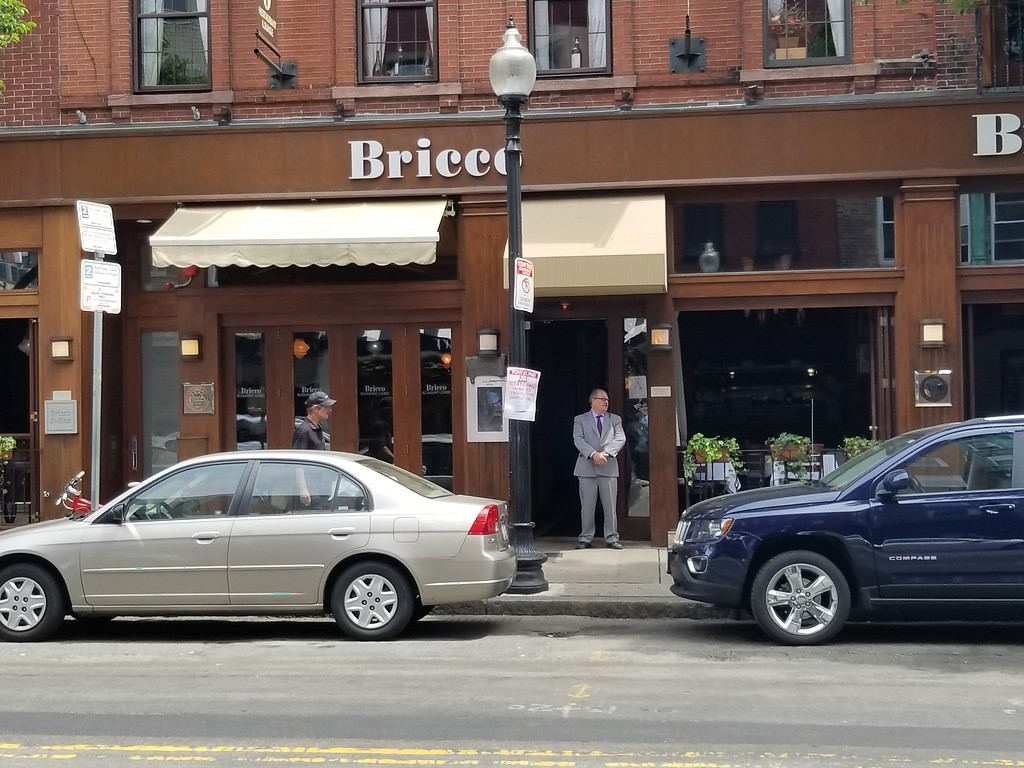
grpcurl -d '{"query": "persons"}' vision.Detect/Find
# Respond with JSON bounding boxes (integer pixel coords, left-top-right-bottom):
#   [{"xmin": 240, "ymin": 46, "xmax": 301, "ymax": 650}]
[
  {"xmin": 291, "ymin": 391, "xmax": 338, "ymax": 512},
  {"xmin": 368, "ymin": 397, "xmax": 394, "ymax": 465},
  {"xmin": 573, "ymin": 389, "xmax": 626, "ymax": 549}
]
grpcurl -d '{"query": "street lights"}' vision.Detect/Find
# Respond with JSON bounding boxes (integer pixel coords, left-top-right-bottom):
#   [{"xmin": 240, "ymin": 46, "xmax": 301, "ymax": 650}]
[{"xmin": 489, "ymin": 13, "xmax": 550, "ymax": 595}]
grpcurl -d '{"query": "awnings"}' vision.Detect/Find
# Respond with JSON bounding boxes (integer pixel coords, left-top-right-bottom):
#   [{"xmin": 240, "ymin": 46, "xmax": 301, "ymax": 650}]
[
  {"xmin": 148, "ymin": 196, "xmax": 448, "ymax": 267},
  {"xmin": 503, "ymin": 192, "xmax": 667, "ymax": 297}
]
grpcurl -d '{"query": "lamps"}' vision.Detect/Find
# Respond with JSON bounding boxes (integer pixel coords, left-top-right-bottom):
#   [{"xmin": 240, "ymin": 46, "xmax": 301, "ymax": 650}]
[
  {"xmin": 684, "ymin": 431, "xmax": 749, "ymax": 477},
  {"xmin": 917, "ymin": 318, "xmax": 946, "ymax": 348},
  {"xmin": 477, "ymin": 327, "xmax": 500, "ymax": 358},
  {"xmin": 648, "ymin": 322, "xmax": 672, "ymax": 351},
  {"xmin": 49, "ymin": 335, "xmax": 73, "ymax": 361},
  {"xmin": 178, "ymin": 332, "xmax": 203, "ymax": 361}
]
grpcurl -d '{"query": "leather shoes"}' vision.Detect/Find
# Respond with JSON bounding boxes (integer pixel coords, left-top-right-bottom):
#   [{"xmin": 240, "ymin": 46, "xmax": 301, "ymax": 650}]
[
  {"xmin": 575, "ymin": 541, "xmax": 592, "ymax": 548},
  {"xmin": 607, "ymin": 541, "xmax": 623, "ymax": 548}
]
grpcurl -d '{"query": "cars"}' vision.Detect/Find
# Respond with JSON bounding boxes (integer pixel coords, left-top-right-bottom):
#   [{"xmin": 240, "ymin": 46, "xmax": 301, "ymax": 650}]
[
  {"xmin": 664, "ymin": 415, "xmax": 1023, "ymax": 647},
  {"xmin": 1, "ymin": 448, "xmax": 520, "ymax": 643},
  {"xmin": 357, "ymin": 433, "xmax": 453, "ymax": 496},
  {"xmin": 150, "ymin": 414, "xmax": 328, "ymax": 476}
]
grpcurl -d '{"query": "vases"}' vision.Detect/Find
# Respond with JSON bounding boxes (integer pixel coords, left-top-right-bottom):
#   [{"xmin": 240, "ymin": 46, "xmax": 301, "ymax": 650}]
[{"xmin": 776, "ymin": 35, "xmax": 799, "ymax": 47}]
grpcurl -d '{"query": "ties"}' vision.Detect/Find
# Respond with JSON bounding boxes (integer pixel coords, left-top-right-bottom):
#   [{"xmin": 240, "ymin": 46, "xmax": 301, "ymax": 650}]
[{"xmin": 596, "ymin": 416, "xmax": 603, "ymax": 438}]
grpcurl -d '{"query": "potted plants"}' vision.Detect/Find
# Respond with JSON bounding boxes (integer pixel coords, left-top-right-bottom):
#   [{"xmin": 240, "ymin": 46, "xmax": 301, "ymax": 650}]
[
  {"xmin": 837, "ymin": 436, "xmax": 880, "ymax": 461},
  {"xmin": 0, "ymin": 435, "xmax": 16, "ymax": 470},
  {"xmin": 764, "ymin": 431, "xmax": 810, "ymax": 485}
]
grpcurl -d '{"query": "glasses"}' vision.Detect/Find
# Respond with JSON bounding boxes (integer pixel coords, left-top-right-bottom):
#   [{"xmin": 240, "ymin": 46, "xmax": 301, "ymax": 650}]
[{"xmin": 594, "ymin": 398, "xmax": 609, "ymax": 401}]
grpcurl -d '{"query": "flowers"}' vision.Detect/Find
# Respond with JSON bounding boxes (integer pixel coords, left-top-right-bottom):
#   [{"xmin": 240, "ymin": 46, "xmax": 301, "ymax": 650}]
[{"xmin": 765, "ymin": 0, "xmax": 813, "ymax": 36}]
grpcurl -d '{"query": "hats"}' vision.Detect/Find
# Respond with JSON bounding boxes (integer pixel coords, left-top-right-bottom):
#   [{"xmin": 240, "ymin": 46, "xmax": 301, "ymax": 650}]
[{"xmin": 308, "ymin": 391, "xmax": 337, "ymax": 406}]
